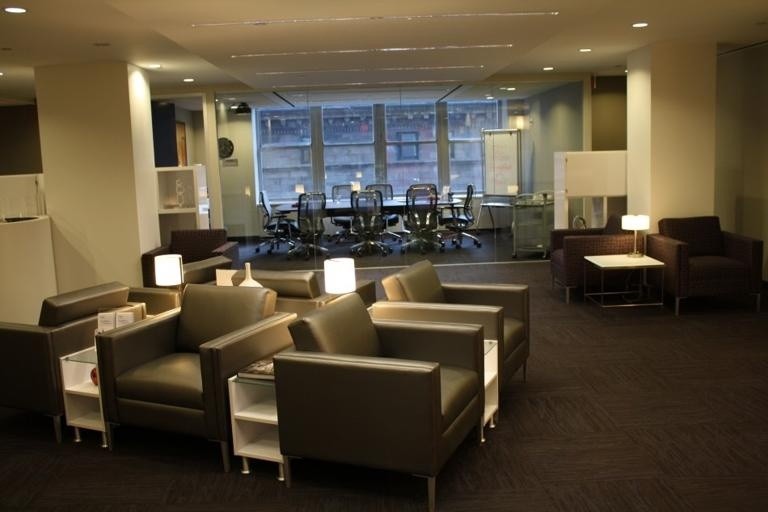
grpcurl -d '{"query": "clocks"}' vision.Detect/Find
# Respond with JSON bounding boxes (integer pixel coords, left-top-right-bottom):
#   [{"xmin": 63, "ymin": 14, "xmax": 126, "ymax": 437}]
[{"xmin": 217, "ymin": 137, "xmax": 233, "ymax": 159}]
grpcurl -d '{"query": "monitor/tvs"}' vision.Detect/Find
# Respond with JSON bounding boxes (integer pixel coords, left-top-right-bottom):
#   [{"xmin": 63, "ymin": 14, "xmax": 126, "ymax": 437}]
[{"xmin": 151, "ymin": 101, "xmax": 178, "ymax": 168}]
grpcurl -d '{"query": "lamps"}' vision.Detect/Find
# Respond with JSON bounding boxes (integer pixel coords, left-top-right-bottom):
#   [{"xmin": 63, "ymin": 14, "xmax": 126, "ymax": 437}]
[
  {"xmin": 228, "ymin": 102, "xmax": 252, "ymax": 115},
  {"xmin": 620, "ymin": 214, "xmax": 649, "ymax": 259}
]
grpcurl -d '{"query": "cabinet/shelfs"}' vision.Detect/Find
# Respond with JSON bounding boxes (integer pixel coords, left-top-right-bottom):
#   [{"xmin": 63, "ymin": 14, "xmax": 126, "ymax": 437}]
[
  {"xmin": 227, "ymin": 358, "xmax": 286, "ymax": 483},
  {"xmin": 153, "ymin": 162, "xmax": 209, "ymax": 248},
  {"xmin": 551, "ymin": 148, "xmax": 626, "ymax": 229},
  {"xmin": 57, "ymin": 345, "xmax": 109, "ymax": 449}
]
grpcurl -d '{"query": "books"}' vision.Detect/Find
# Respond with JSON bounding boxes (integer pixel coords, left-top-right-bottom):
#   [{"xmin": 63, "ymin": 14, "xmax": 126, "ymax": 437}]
[{"xmin": 236, "ymin": 359, "xmax": 275, "ymax": 381}]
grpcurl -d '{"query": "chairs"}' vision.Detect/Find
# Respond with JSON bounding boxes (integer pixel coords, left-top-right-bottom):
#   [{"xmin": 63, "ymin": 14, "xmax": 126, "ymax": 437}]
[{"xmin": 254, "ymin": 177, "xmax": 482, "ymax": 258}]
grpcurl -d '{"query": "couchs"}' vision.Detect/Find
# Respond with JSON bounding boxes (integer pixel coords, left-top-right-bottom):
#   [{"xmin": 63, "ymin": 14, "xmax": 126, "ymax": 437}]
[
  {"xmin": 366, "ymin": 257, "xmax": 530, "ymax": 396},
  {"xmin": 645, "ymin": 215, "xmax": 763, "ymax": 318},
  {"xmin": 0, "ymin": 280, "xmax": 150, "ymax": 452},
  {"xmin": 268, "ymin": 290, "xmax": 489, "ymax": 494},
  {"xmin": 543, "ymin": 212, "xmax": 643, "ymax": 305},
  {"xmin": 215, "ymin": 265, "xmax": 380, "ymax": 315},
  {"xmin": 117, "ymin": 252, "xmax": 235, "ymax": 319},
  {"xmin": 93, "ymin": 282, "xmax": 300, "ymax": 481}
]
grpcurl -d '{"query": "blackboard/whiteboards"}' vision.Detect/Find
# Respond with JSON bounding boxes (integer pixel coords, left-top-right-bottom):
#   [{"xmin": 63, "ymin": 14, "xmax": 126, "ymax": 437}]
[{"xmin": 480, "ymin": 127, "xmax": 521, "ymax": 197}]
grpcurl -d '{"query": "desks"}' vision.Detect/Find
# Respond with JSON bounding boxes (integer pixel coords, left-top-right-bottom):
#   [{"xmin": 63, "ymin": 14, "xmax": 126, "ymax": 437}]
[{"xmin": 582, "ymin": 252, "xmax": 665, "ymax": 311}]
